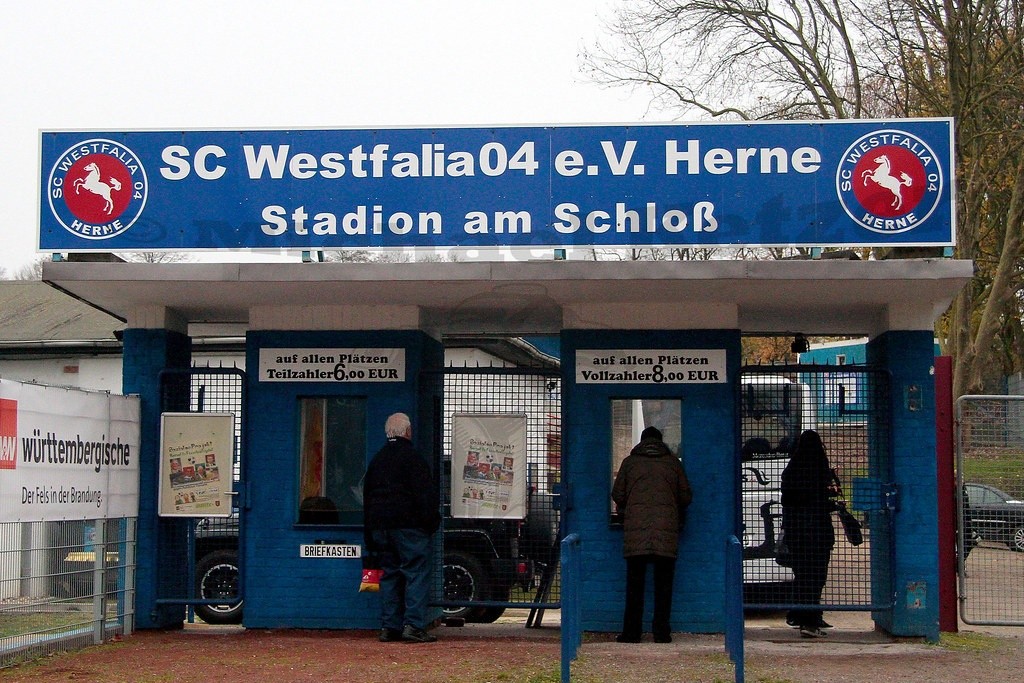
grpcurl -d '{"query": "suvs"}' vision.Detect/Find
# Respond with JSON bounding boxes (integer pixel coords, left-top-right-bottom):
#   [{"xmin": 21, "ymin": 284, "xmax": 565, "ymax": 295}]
[{"xmin": 190, "ymin": 452, "xmax": 536, "ymax": 630}]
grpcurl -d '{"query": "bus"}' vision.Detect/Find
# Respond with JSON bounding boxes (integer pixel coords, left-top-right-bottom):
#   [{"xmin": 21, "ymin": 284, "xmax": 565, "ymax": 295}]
[{"xmin": 632, "ymin": 374, "xmax": 814, "ymax": 589}]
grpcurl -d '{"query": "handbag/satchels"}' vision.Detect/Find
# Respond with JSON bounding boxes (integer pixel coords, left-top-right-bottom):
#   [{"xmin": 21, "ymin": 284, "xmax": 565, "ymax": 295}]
[
  {"xmin": 839, "ymin": 500, "xmax": 862, "ymax": 546},
  {"xmin": 775, "ymin": 536, "xmax": 792, "ymax": 568}
]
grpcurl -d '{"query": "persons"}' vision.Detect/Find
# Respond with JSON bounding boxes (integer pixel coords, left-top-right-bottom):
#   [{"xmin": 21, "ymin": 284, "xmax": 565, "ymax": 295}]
[
  {"xmin": 954, "ymin": 468, "xmax": 973, "ymax": 578},
  {"xmin": 364, "ymin": 413, "xmax": 440, "ymax": 643},
  {"xmin": 170, "ymin": 455, "xmax": 220, "ymax": 483},
  {"xmin": 613, "ymin": 424, "xmax": 691, "ymax": 643},
  {"xmin": 781, "ymin": 430, "xmax": 836, "ymax": 637},
  {"xmin": 465, "ymin": 451, "xmax": 513, "ymax": 486}
]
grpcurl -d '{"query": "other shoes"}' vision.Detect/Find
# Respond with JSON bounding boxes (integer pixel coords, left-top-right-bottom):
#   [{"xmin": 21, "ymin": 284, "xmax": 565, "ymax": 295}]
[
  {"xmin": 656, "ymin": 635, "xmax": 671, "ymax": 643},
  {"xmin": 402, "ymin": 624, "xmax": 437, "ymax": 642},
  {"xmin": 799, "ymin": 626, "xmax": 828, "ymax": 639},
  {"xmin": 617, "ymin": 635, "xmax": 640, "ymax": 643},
  {"xmin": 379, "ymin": 627, "xmax": 409, "ymax": 642}
]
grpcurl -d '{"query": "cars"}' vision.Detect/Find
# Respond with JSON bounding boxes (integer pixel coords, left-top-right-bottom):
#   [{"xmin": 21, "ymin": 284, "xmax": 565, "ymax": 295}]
[{"xmin": 965, "ymin": 482, "xmax": 1024, "ymax": 554}]
